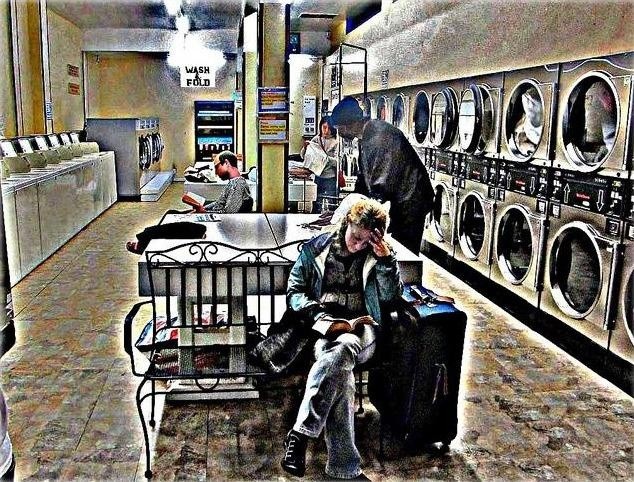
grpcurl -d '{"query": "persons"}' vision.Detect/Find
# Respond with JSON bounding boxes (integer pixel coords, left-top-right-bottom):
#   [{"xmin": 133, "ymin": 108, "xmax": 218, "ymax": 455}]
[
  {"xmin": 246, "ymin": 196, "xmax": 405, "ymax": 482},
  {"xmin": 331, "ymin": 96, "xmax": 436, "ymax": 259},
  {"xmin": 125, "ymin": 149, "xmax": 252, "ymax": 255},
  {"xmin": 299, "ymin": 116, "xmax": 339, "ymax": 214}
]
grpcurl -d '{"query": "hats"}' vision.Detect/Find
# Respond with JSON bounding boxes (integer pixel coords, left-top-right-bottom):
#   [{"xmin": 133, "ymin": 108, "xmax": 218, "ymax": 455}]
[
  {"xmin": 329, "ymin": 95, "xmax": 364, "ymax": 127},
  {"xmin": 280, "ymin": 429, "xmax": 307, "ymax": 478}
]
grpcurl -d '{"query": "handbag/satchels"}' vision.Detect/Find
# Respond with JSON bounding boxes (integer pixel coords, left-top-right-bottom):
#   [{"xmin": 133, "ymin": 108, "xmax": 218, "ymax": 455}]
[
  {"xmin": 335, "ymin": 167, "xmax": 347, "ymax": 190},
  {"xmin": 249, "ymin": 322, "xmax": 314, "ymax": 378}
]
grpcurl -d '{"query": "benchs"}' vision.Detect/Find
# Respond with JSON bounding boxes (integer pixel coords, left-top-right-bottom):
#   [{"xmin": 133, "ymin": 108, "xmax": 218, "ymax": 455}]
[{"xmin": 122, "ymin": 236, "xmax": 399, "ymax": 479}]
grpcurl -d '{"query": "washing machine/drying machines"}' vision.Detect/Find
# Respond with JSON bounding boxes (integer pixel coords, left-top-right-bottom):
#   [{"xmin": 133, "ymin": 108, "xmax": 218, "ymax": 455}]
[
  {"xmin": 0, "ymin": 117, "xmax": 165, "ymax": 290},
  {"xmin": 342, "ymin": 52, "xmax": 634, "ymax": 399}
]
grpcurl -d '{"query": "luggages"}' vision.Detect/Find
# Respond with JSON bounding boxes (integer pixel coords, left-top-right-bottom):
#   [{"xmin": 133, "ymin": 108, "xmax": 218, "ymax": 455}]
[{"xmin": 365, "ymin": 280, "xmax": 469, "ymax": 453}]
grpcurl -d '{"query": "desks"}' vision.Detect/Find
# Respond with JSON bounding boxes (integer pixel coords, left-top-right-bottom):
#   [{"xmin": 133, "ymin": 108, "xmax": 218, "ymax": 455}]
[
  {"xmin": 136, "ymin": 210, "xmax": 424, "ymax": 406},
  {"xmin": 183, "ymin": 161, "xmax": 317, "ymax": 214}
]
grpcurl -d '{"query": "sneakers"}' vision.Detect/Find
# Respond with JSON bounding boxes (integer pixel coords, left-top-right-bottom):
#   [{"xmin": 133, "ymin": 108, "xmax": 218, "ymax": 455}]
[{"xmin": 126, "ymin": 240, "xmax": 144, "ymax": 255}]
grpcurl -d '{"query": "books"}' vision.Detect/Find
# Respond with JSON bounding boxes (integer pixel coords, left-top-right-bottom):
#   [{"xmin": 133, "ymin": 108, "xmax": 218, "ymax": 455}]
[
  {"xmin": 310, "ymin": 310, "xmax": 379, "ymax": 335},
  {"xmin": 180, "ymin": 190, "xmax": 207, "ymax": 208}
]
